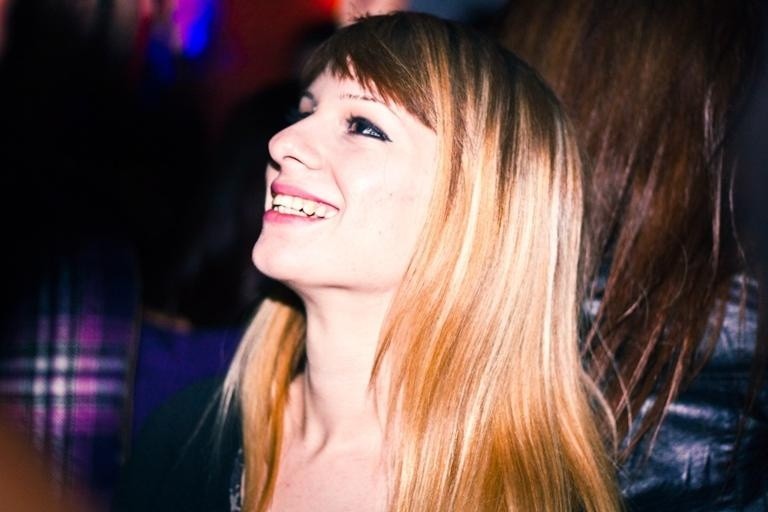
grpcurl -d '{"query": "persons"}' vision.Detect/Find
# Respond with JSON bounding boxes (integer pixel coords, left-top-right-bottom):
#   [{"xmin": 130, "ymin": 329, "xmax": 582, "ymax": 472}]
[
  {"xmin": 478, "ymin": 0, "xmax": 767, "ymax": 512},
  {"xmin": 123, "ymin": 11, "xmax": 628, "ymax": 512}
]
